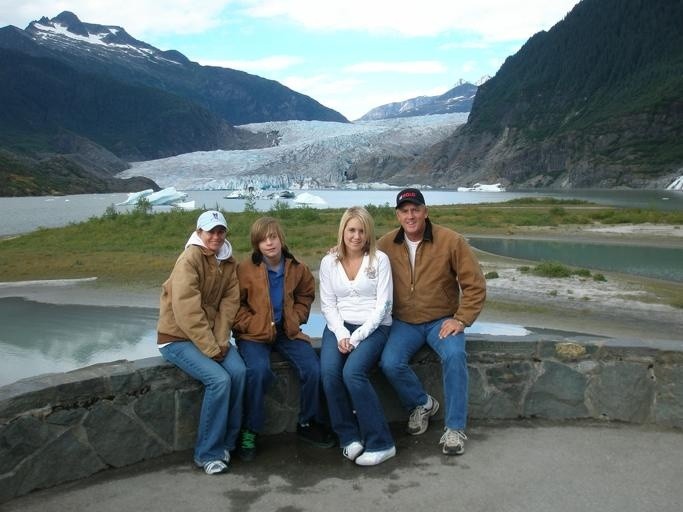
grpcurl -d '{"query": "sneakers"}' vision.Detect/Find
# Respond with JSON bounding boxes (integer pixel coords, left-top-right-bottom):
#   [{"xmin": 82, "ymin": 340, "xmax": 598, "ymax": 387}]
[
  {"xmin": 344, "ymin": 439, "xmax": 396, "ymax": 466},
  {"xmin": 195, "ymin": 449, "xmax": 231, "ymax": 475},
  {"xmin": 442, "ymin": 426, "xmax": 466, "ymax": 455},
  {"xmin": 239, "ymin": 428, "xmax": 257, "ymax": 462},
  {"xmin": 297, "ymin": 423, "xmax": 336, "ymax": 448},
  {"xmin": 408, "ymin": 395, "xmax": 439, "ymax": 435}
]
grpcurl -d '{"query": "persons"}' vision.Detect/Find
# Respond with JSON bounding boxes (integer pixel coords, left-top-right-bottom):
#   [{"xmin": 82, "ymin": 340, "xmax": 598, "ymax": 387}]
[
  {"xmin": 158, "ymin": 210, "xmax": 246, "ymax": 475},
  {"xmin": 326, "ymin": 188, "xmax": 487, "ymax": 455},
  {"xmin": 319, "ymin": 206, "xmax": 397, "ymax": 467},
  {"xmin": 234, "ymin": 216, "xmax": 336, "ymax": 464}
]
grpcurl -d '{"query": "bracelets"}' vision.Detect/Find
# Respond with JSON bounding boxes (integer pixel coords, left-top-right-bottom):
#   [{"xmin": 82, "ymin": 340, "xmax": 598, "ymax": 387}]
[{"xmin": 455, "ymin": 319, "xmax": 467, "ymax": 328}]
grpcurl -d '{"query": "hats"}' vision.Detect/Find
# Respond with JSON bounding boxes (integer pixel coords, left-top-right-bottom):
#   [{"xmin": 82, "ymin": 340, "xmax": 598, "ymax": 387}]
[
  {"xmin": 395, "ymin": 188, "xmax": 425, "ymax": 208},
  {"xmin": 197, "ymin": 211, "xmax": 229, "ymax": 232}
]
